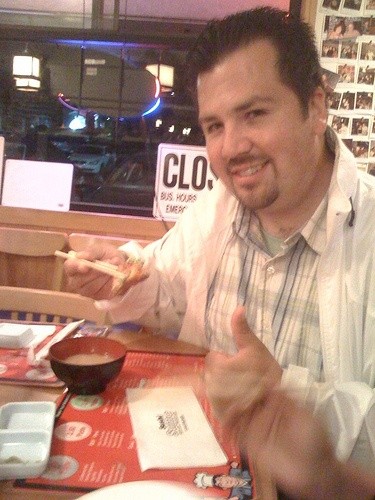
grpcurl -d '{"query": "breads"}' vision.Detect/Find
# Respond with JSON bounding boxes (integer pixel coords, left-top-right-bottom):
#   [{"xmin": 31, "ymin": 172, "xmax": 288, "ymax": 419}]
[{"xmin": 111, "ymin": 257, "xmax": 149, "ymax": 296}]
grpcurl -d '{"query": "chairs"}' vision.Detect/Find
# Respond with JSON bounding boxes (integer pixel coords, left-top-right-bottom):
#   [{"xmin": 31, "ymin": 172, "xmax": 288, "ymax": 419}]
[{"xmin": 0, "ymin": 227, "xmax": 153, "ymax": 321}]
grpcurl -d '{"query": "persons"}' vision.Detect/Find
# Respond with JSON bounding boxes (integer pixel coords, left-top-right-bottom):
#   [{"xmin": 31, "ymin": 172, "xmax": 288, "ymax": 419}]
[
  {"xmin": 238, "ymin": 388, "xmax": 375, "ymax": 500},
  {"xmin": 321, "ymin": 0, "xmax": 375, "ymax": 159},
  {"xmin": 64, "ymin": 5, "xmax": 375, "ymax": 500}
]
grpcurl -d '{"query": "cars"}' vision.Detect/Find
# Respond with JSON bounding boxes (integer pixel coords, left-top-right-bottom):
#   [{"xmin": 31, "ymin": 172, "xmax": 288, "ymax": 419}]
[
  {"xmin": 82, "ymin": 151, "xmax": 157, "ymax": 217},
  {"xmin": 66, "ymin": 142, "xmax": 118, "ymax": 183}
]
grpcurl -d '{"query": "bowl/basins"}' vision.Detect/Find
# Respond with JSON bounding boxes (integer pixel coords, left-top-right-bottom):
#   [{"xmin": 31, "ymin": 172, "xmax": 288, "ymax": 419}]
[{"xmin": 48, "ymin": 337, "xmax": 128, "ymax": 396}]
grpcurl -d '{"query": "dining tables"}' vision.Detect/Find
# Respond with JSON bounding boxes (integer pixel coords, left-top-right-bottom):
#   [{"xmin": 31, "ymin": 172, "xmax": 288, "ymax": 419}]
[{"xmin": 0, "ymin": 319, "xmax": 277, "ymax": 500}]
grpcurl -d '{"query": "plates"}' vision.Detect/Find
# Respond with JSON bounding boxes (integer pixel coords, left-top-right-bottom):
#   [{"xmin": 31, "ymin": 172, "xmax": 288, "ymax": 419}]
[
  {"xmin": 76, "ymin": 481, "xmax": 204, "ymax": 499},
  {"xmin": 0, "ymin": 401, "xmax": 56, "ymax": 479}
]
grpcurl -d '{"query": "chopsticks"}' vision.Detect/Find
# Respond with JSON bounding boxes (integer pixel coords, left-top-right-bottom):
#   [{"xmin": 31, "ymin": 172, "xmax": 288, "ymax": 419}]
[{"xmin": 54, "ymin": 250, "xmax": 124, "ymax": 280}]
